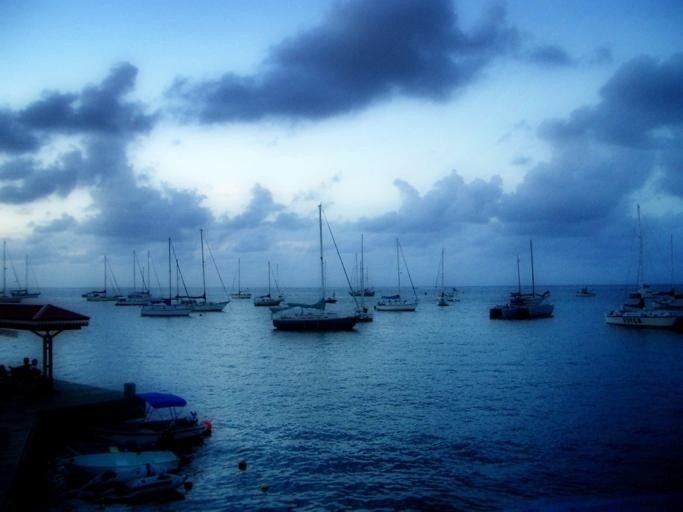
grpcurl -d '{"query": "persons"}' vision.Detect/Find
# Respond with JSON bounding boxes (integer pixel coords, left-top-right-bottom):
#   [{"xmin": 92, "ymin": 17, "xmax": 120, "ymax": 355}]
[{"xmin": 0, "ymin": 356, "xmax": 42, "ymax": 377}]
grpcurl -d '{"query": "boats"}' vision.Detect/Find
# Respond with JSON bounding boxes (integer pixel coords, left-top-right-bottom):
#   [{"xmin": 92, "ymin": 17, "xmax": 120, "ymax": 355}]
[
  {"xmin": 67, "ymin": 448, "xmax": 182, "ymax": 487},
  {"xmin": 100, "ymin": 389, "xmax": 215, "ymax": 452}
]
